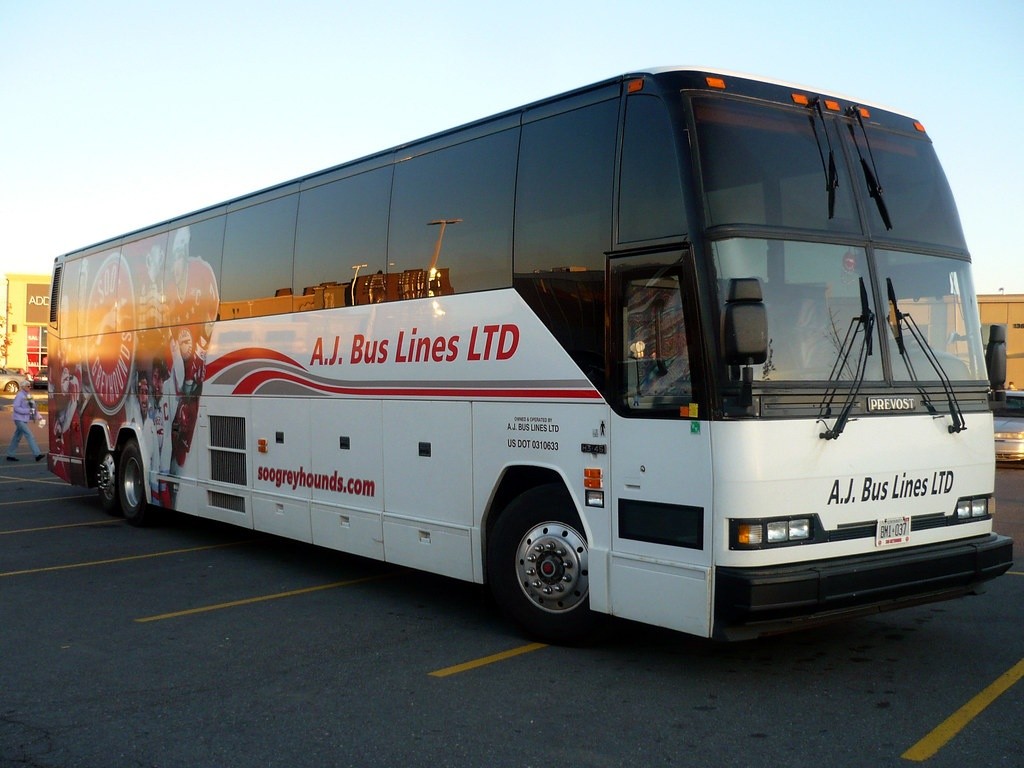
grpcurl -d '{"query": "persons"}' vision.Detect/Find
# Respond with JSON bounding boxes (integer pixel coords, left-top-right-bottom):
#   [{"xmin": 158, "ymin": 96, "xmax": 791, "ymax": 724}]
[
  {"xmin": 1007, "ymin": 382, "xmax": 1018, "ymax": 391},
  {"xmin": 6, "ymin": 380, "xmax": 46, "ymax": 462},
  {"xmin": 53, "ymin": 225, "xmax": 218, "ymax": 508}
]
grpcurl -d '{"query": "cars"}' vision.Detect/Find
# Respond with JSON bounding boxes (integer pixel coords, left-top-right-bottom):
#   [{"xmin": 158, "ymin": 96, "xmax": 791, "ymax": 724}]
[
  {"xmin": 0, "ymin": 367, "xmax": 33, "ymax": 393},
  {"xmin": 987, "ymin": 390, "xmax": 1024, "ymax": 465},
  {"xmin": 33, "ymin": 368, "xmax": 48, "ymax": 388}
]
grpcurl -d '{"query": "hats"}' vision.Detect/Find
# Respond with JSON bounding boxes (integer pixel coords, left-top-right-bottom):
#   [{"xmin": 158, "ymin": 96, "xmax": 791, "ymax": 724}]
[{"xmin": 19, "ymin": 380, "xmax": 30, "ymax": 387}]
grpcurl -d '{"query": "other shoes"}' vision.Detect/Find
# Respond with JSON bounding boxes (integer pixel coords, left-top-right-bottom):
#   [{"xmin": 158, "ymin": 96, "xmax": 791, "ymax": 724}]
[
  {"xmin": 36, "ymin": 454, "xmax": 46, "ymax": 461},
  {"xmin": 7, "ymin": 456, "xmax": 19, "ymax": 461}
]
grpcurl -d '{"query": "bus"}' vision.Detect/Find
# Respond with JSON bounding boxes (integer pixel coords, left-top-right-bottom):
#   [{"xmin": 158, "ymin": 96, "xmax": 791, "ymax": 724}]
[{"xmin": 47, "ymin": 64, "xmax": 1018, "ymax": 643}]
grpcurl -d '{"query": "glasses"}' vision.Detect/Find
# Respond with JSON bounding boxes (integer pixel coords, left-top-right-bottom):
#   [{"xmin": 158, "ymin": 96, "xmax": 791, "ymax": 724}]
[{"xmin": 27, "ymin": 385, "xmax": 30, "ymax": 388}]
